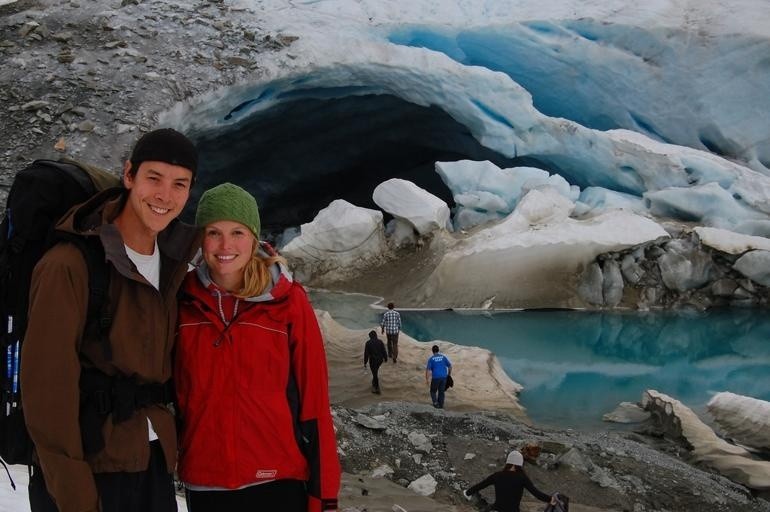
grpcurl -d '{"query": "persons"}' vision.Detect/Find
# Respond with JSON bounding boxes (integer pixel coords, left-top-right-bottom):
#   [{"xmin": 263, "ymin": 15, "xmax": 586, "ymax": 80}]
[
  {"xmin": 381, "ymin": 303, "xmax": 401, "ymax": 363},
  {"xmin": 363, "ymin": 330, "xmax": 388, "ymax": 395},
  {"xmin": 464, "ymin": 451, "xmax": 558, "ymax": 512},
  {"xmin": 425, "ymin": 345, "xmax": 452, "ymax": 409},
  {"xmin": 175, "ymin": 182, "xmax": 341, "ymax": 512},
  {"xmin": 18, "ymin": 127, "xmax": 205, "ymax": 512}
]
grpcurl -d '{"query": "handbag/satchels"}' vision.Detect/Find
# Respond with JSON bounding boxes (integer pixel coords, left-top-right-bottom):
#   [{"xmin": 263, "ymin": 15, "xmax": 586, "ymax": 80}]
[{"xmin": 544, "ymin": 491, "xmax": 569, "ymax": 512}]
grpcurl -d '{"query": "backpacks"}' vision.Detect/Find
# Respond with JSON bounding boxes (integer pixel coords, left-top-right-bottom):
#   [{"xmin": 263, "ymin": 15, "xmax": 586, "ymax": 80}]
[{"xmin": 0, "ymin": 159, "xmax": 172, "ymax": 470}]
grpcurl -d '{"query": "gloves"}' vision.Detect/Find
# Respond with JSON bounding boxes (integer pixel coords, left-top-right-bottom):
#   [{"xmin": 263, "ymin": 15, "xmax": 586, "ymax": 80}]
[{"xmin": 463, "ymin": 490, "xmax": 473, "ymax": 501}]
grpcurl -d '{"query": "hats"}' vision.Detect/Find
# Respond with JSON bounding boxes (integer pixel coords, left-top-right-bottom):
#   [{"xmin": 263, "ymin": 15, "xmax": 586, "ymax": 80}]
[
  {"xmin": 194, "ymin": 183, "xmax": 260, "ymax": 238},
  {"xmin": 131, "ymin": 128, "xmax": 198, "ymax": 173},
  {"xmin": 506, "ymin": 451, "xmax": 524, "ymax": 466}
]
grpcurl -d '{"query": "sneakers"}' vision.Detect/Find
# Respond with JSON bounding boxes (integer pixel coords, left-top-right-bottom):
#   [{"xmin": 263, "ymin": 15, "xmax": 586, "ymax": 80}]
[
  {"xmin": 388, "ymin": 352, "xmax": 397, "ymax": 363},
  {"xmin": 372, "ymin": 387, "xmax": 380, "ymax": 394}
]
